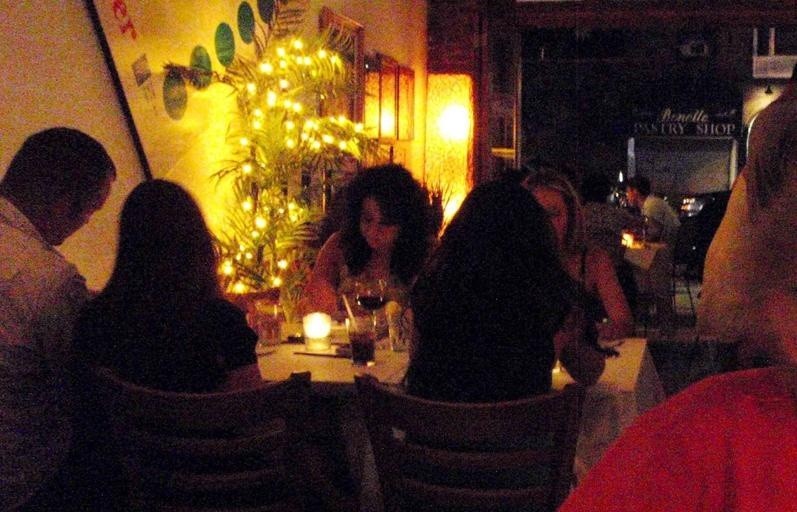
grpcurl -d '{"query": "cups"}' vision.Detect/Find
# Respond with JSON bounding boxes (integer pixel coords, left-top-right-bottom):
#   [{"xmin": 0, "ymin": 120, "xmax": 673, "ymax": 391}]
[
  {"xmin": 302, "ymin": 312, "xmax": 333, "ymax": 353},
  {"xmin": 344, "ymin": 313, "xmax": 377, "ymax": 369}
]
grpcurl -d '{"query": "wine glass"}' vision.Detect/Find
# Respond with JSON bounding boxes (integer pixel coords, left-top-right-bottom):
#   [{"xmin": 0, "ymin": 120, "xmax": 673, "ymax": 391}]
[{"xmin": 351, "ymin": 278, "xmax": 385, "ymax": 317}]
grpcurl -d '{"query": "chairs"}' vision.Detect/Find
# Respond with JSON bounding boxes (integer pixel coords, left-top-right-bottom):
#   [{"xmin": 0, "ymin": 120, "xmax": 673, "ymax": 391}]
[
  {"xmin": 672, "ymin": 234, "xmax": 699, "ymax": 319},
  {"xmin": 352, "ymin": 373, "xmax": 587, "ymax": 512},
  {"xmin": 94, "ymin": 371, "xmax": 312, "ymax": 511}
]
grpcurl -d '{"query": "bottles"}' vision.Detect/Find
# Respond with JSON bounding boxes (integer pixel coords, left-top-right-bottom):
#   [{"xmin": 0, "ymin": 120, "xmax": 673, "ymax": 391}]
[{"xmin": 681, "ymin": 198, "xmax": 700, "ymax": 217}]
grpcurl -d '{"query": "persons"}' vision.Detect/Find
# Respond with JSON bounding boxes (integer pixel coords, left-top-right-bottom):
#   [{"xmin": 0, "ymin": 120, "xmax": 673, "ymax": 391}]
[
  {"xmin": 410, "ymin": 181, "xmax": 605, "ymax": 511},
  {"xmin": 307, "ymin": 166, "xmax": 440, "ymax": 323},
  {"xmin": 627, "ymin": 175, "xmax": 682, "ymax": 266},
  {"xmin": 77, "ymin": 180, "xmax": 261, "ymax": 511},
  {"xmin": 523, "ymin": 168, "xmax": 635, "ymax": 339},
  {"xmin": 555, "ymin": 75, "xmax": 796, "ymax": 511},
  {"xmin": 576, "ymin": 182, "xmax": 642, "ymax": 321},
  {"xmin": 0, "ymin": 125, "xmax": 117, "ymax": 505},
  {"xmin": 609, "ymin": 183, "xmax": 624, "ymax": 206}
]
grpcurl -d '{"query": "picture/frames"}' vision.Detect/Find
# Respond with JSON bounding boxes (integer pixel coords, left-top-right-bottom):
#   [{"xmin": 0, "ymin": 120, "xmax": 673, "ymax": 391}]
[
  {"xmin": 361, "ymin": 55, "xmax": 379, "ymax": 141},
  {"xmin": 397, "ymin": 66, "xmax": 415, "ymax": 142},
  {"xmin": 318, "ymin": 5, "xmax": 367, "ymax": 131},
  {"xmin": 376, "ymin": 51, "xmax": 398, "ymax": 143}
]
